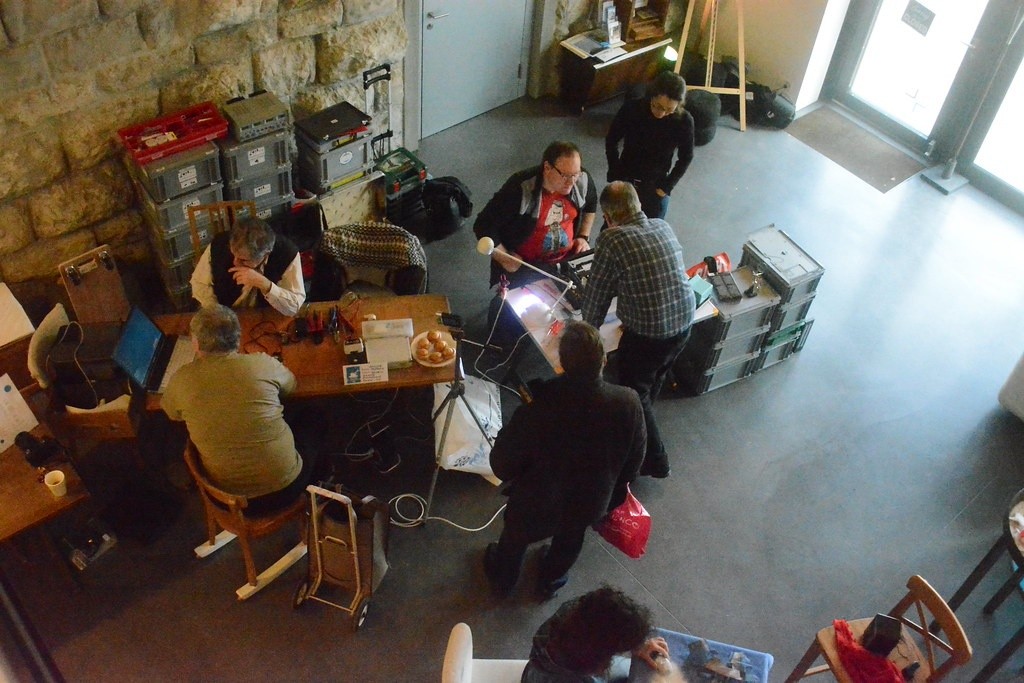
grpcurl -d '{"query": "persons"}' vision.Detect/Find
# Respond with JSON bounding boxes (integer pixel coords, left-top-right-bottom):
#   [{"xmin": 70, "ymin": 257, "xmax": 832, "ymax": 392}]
[
  {"xmin": 473, "ymin": 141, "xmax": 599, "ymax": 348},
  {"xmin": 600, "ymin": 73, "xmax": 696, "ymax": 231},
  {"xmin": 520, "ymin": 583, "xmax": 669, "ymax": 683},
  {"xmin": 483, "ymin": 320, "xmax": 649, "ymax": 598},
  {"xmin": 581, "ymin": 179, "xmax": 695, "ymax": 480},
  {"xmin": 159, "ymin": 304, "xmax": 342, "ymax": 518},
  {"xmin": 189, "ymin": 217, "xmax": 306, "ymax": 318}
]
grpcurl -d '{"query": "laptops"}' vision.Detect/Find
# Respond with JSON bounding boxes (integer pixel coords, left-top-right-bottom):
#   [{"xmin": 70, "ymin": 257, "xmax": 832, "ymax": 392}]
[{"xmin": 110, "ymin": 302, "xmax": 197, "ymax": 394}]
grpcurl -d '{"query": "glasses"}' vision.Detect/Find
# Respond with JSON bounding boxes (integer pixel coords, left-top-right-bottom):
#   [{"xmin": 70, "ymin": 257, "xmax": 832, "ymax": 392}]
[
  {"xmin": 652, "ymin": 96, "xmax": 678, "ymax": 114},
  {"xmin": 550, "ymin": 163, "xmax": 583, "ymax": 181}
]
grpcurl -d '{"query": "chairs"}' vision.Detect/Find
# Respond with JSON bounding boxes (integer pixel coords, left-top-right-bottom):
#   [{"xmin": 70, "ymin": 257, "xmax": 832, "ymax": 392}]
[
  {"xmin": 27, "ymin": 302, "xmax": 143, "ymax": 453},
  {"xmin": 439, "ymin": 623, "xmax": 528, "ymax": 683},
  {"xmin": 783, "ymin": 575, "xmax": 973, "ymax": 683},
  {"xmin": 181, "ymin": 436, "xmax": 310, "ymax": 601}
]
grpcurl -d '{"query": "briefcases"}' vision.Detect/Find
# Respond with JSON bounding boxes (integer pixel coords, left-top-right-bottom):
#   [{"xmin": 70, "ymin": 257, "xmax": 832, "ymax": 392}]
[{"xmin": 294, "ymin": 101, "xmax": 373, "ymax": 155}]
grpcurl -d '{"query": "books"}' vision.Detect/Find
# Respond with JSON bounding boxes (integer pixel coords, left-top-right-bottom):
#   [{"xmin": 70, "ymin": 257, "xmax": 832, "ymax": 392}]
[{"xmin": 559, "ymin": 0, "xmax": 627, "ymax": 59}]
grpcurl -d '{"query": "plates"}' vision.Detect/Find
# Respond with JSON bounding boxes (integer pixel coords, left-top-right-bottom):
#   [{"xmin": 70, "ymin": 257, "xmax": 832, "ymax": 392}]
[{"xmin": 410, "ymin": 329, "xmax": 457, "ymax": 368}]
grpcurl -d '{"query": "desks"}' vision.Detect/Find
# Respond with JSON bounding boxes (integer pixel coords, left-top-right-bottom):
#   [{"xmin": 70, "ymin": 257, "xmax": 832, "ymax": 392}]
[
  {"xmin": 142, "ymin": 288, "xmax": 467, "ymax": 468},
  {"xmin": 499, "ymin": 271, "xmax": 719, "ymax": 391},
  {"xmin": 0, "ymin": 415, "xmax": 93, "ymax": 683},
  {"xmin": 626, "ymin": 627, "xmax": 776, "ymax": 683}
]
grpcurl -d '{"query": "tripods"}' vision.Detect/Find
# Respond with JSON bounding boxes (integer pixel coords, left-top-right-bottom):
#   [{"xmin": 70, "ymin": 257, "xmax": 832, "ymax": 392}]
[{"xmin": 393, "ymin": 327, "xmax": 494, "ymax": 529}]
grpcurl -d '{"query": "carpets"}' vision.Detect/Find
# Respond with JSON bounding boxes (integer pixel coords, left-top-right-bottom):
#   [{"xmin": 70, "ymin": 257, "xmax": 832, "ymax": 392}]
[{"xmin": 786, "ymin": 103, "xmax": 926, "ymax": 194}]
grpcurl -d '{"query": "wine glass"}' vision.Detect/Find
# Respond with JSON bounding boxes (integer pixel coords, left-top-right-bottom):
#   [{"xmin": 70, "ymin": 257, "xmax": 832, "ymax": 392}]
[{"xmin": 751, "ymin": 264, "xmax": 764, "ymax": 291}]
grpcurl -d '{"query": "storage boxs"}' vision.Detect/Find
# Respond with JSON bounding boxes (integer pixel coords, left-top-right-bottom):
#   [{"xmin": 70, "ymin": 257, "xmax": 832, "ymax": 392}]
[
  {"xmin": 670, "ymin": 228, "xmax": 825, "ymax": 397},
  {"xmin": 115, "ymin": 85, "xmax": 430, "ymax": 316}
]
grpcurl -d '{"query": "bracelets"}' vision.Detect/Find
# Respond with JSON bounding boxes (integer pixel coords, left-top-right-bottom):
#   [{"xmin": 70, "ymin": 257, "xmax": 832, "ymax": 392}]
[{"xmin": 576, "ymin": 235, "xmax": 590, "ymax": 243}]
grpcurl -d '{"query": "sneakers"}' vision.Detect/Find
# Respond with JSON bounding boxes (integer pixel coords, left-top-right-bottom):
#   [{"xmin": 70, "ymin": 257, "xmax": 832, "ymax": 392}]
[
  {"xmin": 484, "ymin": 542, "xmax": 513, "ymax": 593},
  {"xmin": 539, "ymin": 544, "xmax": 560, "ymax": 598}
]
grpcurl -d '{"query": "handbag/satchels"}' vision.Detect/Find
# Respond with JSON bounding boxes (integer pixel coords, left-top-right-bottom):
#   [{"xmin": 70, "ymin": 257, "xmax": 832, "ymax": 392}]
[{"xmin": 592, "ymin": 482, "xmax": 652, "ymax": 558}]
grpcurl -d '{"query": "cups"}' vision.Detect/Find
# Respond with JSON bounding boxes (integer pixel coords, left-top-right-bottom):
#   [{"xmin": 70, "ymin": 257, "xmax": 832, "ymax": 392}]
[{"xmin": 44, "ymin": 469, "xmax": 67, "ymax": 497}]
